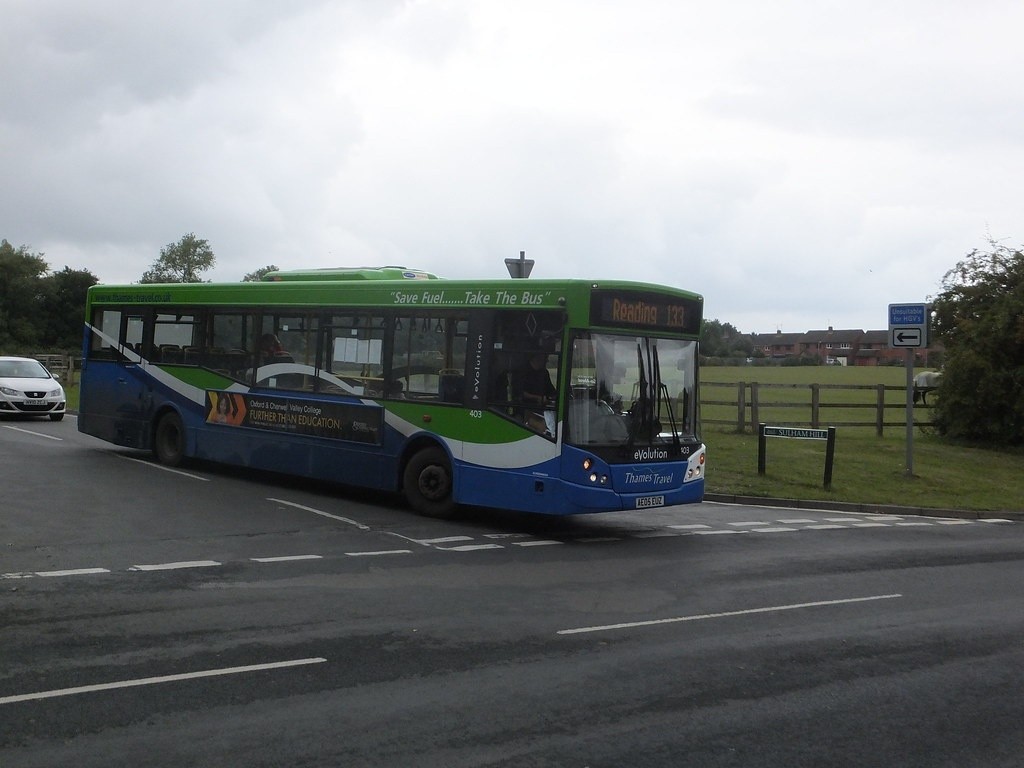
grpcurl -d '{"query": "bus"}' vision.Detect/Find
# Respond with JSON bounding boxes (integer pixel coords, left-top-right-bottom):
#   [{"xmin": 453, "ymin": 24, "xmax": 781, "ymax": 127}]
[{"xmin": 76, "ymin": 266, "xmax": 706, "ymax": 519}]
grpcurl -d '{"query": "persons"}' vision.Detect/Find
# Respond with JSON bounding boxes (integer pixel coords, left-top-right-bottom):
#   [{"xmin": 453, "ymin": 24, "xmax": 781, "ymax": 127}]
[
  {"xmin": 515, "ymin": 354, "xmax": 556, "ymax": 410},
  {"xmin": 236, "ymin": 333, "xmax": 278, "ymax": 382},
  {"xmin": 389, "ymin": 381, "xmax": 405, "ymax": 398}
]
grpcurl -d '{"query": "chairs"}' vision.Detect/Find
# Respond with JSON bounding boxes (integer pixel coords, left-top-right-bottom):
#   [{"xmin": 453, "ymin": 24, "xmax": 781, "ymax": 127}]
[{"xmin": 115, "ymin": 341, "xmax": 591, "ymax": 422}]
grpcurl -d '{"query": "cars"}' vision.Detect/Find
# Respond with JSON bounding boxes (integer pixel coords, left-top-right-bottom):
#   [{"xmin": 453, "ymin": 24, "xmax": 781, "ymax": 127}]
[{"xmin": 0, "ymin": 356, "xmax": 66, "ymax": 421}]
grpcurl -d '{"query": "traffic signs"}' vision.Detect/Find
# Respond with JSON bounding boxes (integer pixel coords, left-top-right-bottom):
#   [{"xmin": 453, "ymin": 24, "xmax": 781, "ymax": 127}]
[{"xmin": 888, "ymin": 303, "xmax": 929, "ymax": 348}]
